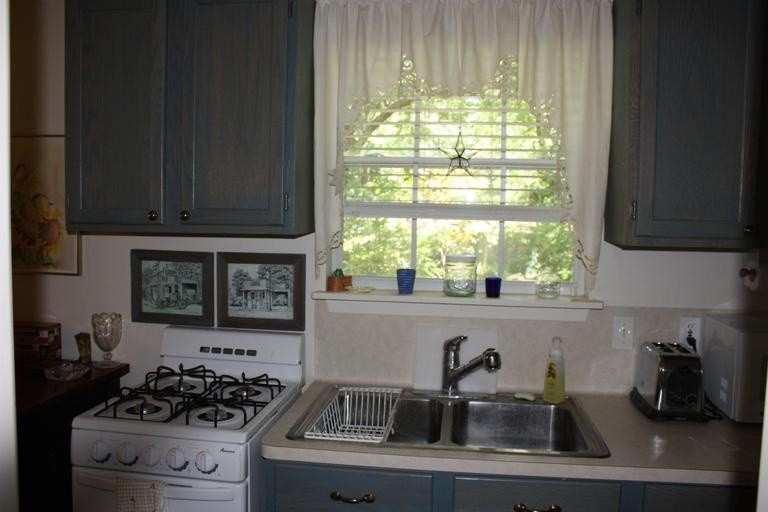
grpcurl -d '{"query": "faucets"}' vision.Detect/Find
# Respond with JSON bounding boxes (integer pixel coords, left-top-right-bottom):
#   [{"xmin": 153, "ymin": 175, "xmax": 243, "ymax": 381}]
[{"xmin": 441, "ymin": 335, "xmax": 501, "ymax": 395}]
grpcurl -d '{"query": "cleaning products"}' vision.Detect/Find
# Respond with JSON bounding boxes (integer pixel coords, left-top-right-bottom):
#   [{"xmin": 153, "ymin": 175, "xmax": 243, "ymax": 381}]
[{"xmin": 542, "ymin": 335, "xmax": 568, "ymax": 404}]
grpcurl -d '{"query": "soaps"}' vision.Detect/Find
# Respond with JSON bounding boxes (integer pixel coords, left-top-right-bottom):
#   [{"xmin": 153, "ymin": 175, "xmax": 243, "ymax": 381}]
[{"xmin": 514, "ymin": 392, "xmax": 538, "ymax": 401}]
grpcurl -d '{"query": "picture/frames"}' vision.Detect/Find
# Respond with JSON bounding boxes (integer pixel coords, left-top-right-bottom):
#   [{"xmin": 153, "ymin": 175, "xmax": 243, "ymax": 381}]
[
  {"xmin": 13, "ymin": 134, "xmax": 81, "ymax": 276},
  {"xmin": 131, "ymin": 248, "xmax": 306, "ymax": 331}
]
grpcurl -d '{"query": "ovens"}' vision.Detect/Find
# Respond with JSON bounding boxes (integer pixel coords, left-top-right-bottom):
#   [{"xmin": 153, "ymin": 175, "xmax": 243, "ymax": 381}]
[{"xmin": 71, "ymin": 428, "xmax": 247, "ymax": 512}]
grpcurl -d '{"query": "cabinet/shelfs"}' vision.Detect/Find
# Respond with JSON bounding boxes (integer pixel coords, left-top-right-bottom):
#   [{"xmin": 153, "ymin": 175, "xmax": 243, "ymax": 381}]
[
  {"xmin": 65, "ymin": 0, "xmax": 313, "ymax": 239},
  {"xmin": 604, "ymin": 1, "xmax": 768, "ymax": 251},
  {"xmin": 262, "ymin": 460, "xmax": 757, "ymax": 511}
]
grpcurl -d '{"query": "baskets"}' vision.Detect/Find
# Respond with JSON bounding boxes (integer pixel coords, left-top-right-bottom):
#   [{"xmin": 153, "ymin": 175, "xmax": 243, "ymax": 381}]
[{"xmin": 304, "ymin": 385, "xmax": 405, "ymax": 443}]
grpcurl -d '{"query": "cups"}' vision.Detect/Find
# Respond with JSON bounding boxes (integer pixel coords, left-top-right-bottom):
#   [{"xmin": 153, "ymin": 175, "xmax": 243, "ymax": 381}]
[
  {"xmin": 485, "ymin": 277, "xmax": 502, "ymax": 299},
  {"xmin": 397, "ymin": 268, "xmax": 416, "ymax": 295}
]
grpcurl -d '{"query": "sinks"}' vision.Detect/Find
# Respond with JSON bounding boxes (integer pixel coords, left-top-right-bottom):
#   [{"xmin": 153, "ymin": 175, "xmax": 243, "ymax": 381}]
[
  {"xmin": 446, "ymin": 392, "xmax": 610, "ymax": 458},
  {"xmin": 285, "ymin": 381, "xmax": 447, "ymax": 450}
]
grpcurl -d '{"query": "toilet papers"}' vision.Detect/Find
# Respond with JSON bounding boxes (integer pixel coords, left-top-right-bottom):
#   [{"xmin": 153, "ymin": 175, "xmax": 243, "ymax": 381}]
[{"xmin": 742, "ymin": 262, "xmax": 761, "ymax": 291}]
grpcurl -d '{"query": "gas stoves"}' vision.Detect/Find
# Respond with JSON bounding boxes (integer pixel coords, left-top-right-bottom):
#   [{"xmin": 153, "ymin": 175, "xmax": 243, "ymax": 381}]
[{"xmin": 70, "ymin": 362, "xmax": 300, "ymax": 444}]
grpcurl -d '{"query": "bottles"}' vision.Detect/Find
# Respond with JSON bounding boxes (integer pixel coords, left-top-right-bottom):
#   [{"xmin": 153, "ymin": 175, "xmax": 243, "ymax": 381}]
[
  {"xmin": 442, "ymin": 254, "xmax": 477, "ymax": 296},
  {"xmin": 542, "ymin": 336, "xmax": 567, "ymax": 404},
  {"xmin": 536, "ymin": 265, "xmax": 560, "ymax": 300}
]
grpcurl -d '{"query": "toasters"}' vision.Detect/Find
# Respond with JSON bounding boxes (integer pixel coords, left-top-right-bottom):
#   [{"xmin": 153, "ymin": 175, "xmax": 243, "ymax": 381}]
[{"xmin": 628, "ymin": 341, "xmax": 709, "ymax": 424}]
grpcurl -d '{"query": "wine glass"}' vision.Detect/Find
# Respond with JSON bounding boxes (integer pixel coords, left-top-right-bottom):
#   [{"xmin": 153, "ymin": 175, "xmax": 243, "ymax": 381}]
[{"xmin": 90, "ymin": 312, "xmax": 123, "ymax": 369}]
